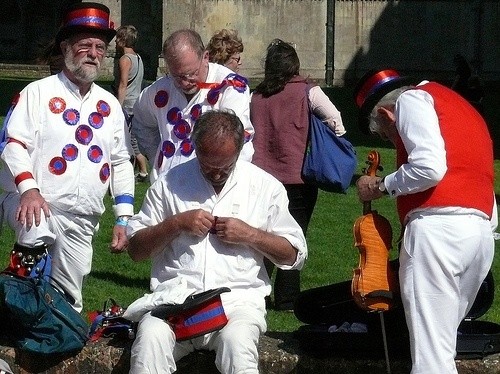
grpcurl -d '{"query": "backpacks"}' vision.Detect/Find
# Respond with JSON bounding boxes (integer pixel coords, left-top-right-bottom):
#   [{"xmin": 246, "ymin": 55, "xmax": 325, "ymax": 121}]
[{"xmin": 0, "ymin": 272, "xmax": 89, "ymax": 357}]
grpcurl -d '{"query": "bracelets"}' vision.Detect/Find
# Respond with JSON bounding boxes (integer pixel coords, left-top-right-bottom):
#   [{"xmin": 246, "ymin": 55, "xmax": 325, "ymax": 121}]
[{"xmin": 116, "ymin": 217, "xmax": 130, "ymax": 225}]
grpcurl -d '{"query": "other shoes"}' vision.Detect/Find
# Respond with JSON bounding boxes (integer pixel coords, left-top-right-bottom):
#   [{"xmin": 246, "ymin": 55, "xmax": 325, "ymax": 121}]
[{"xmin": 134, "ymin": 172, "xmax": 150, "ymax": 181}]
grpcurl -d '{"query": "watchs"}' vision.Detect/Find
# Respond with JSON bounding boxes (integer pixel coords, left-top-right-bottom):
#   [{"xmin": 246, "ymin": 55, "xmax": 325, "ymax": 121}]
[{"xmin": 378, "ymin": 176, "xmax": 391, "ymax": 194}]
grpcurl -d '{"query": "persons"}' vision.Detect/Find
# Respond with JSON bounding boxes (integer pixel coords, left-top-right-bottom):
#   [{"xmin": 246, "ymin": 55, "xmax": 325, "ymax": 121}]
[
  {"xmin": 0, "ymin": 0, "xmax": 134, "ymax": 312},
  {"xmin": 249, "ymin": 38, "xmax": 348, "ymax": 311},
  {"xmin": 126, "ymin": 109, "xmax": 310, "ymax": 374},
  {"xmin": 343, "ymin": 56, "xmax": 499, "ymax": 374},
  {"xmin": 114, "ymin": 25, "xmax": 150, "ymax": 180},
  {"xmin": 128, "ymin": 29, "xmax": 255, "ymax": 185},
  {"xmin": 206, "ymin": 30, "xmax": 243, "ymax": 74}
]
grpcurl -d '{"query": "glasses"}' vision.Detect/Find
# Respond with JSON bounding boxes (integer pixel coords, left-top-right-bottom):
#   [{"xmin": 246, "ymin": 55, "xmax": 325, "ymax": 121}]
[
  {"xmin": 73, "ymin": 40, "xmax": 106, "ymax": 55},
  {"xmin": 229, "ymin": 56, "xmax": 240, "ymax": 63},
  {"xmin": 169, "ymin": 55, "xmax": 205, "ymax": 80}
]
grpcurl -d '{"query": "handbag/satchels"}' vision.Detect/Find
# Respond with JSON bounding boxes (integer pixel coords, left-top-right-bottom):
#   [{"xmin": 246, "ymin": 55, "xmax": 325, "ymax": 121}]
[{"xmin": 301, "ymin": 83, "xmax": 357, "ymax": 194}]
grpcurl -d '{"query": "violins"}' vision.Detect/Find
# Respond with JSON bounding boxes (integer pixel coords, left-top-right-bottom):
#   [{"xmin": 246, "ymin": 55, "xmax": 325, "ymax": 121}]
[{"xmin": 351, "ymin": 150, "xmax": 395, "ymax": 314}]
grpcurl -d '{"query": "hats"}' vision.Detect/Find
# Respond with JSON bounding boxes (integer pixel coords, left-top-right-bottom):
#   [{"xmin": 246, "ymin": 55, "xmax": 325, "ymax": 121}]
[
  {"xmin": 356, "ymin": 70, "xmax": 418, "ymax": 137},
  {"xmin": 56, "ymin": 2, "xmax": 117, "ymax": 50},
  {"xmin": 150, "ymin": 287, "xmax": 231, "ymax": 341}
]
